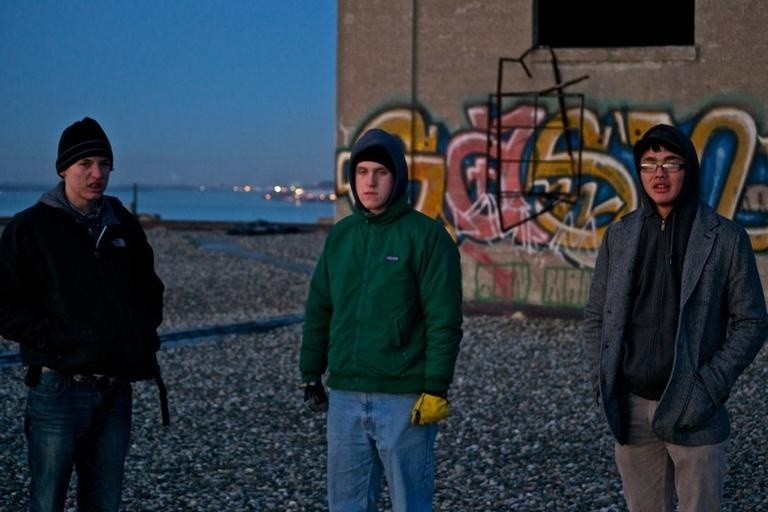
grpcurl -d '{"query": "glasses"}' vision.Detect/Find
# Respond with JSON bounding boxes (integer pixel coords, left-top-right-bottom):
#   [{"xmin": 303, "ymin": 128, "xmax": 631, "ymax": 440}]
[{"xmin": 639, "ymin": 161, "xmax": 685, "ymax": 173}]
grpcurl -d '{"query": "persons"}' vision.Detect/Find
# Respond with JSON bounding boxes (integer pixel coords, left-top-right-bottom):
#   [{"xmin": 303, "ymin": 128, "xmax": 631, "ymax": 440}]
[
  {"xmin": 0, "ymin": 117, "xmax": 166, "ymax": 512},
  {"xmin": 582, "ymin": 124, "xmax": 768, "ymax": 512},
  {"xmin": 298, "ymin": 128, "xmax": 464, "ymax": 512}
]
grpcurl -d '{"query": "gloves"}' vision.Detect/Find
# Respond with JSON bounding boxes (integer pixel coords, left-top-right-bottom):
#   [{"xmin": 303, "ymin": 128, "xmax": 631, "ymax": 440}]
[
  {"xmin": 303, "ymin": 376, "xmax": 328, "ymax": 412},
  {"xmin": 410, "ymin": 391, "xmax": 451, "ymax": 426}
]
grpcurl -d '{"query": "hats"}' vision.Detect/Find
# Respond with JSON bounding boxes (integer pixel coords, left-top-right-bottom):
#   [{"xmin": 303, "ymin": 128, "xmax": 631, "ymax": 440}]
[
  {"xmin": 56, "ymin": 116, "xmax": 114, "ymax": 180},
  {"xmin": 352, "ymin": 144, "xmax": 395, "ymax": 178}
]
